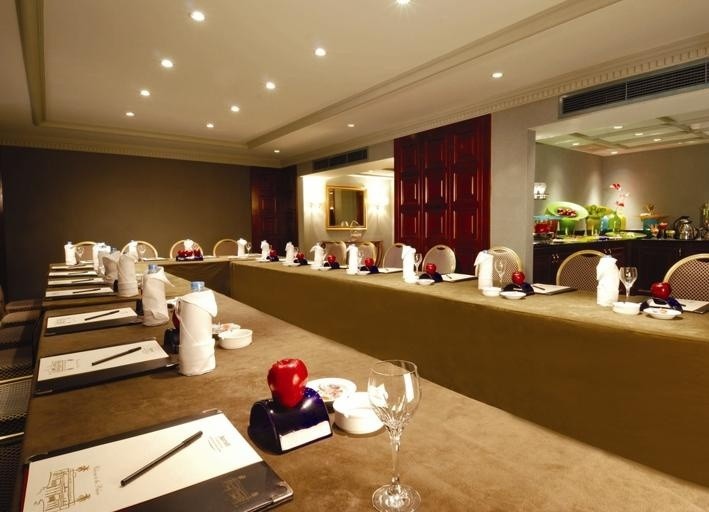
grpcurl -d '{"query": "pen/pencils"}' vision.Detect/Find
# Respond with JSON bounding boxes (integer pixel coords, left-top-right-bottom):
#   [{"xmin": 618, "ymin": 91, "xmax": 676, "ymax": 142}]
[
  {"xmin": 69, "ymin": 264, "xmax": 94, "ymax": 283},
  {"xmin": 92, "ymin": 347, "xmax": 141, "ymax": 365},
  {"xmin": 445, "ymin": 274, "xmax": 452, "ymax": 279},
  {"xmin": 85, "ymin": 310, "xmax": 119, "ymax": 321},
  {"xmin": 73, "ymin": 289, "xmax": 100, "ymax": 293},
  {"xmin": 121, "ymin": 431, "xmax": 202, "ymax": 486},
  {"xmin": 533, "ymin": 286, "xmax": 545, "ymax": 291}
]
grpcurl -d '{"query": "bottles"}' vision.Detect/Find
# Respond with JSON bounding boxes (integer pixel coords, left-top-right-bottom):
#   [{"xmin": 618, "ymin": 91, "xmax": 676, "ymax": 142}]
[
  {"xmin": 190, "ymin": 280, "xmax": 206, "ymax": 294},
  {"xmin": 482, "ymin": 249, "xmax": 488, "ymax": 254},
  {"xmin": 66, "ymin": 240, "xmax": 147, "ymax": 258},
  {"xmin": 147, "ymin": 264, "xmax": 157, "ymax": 273}
]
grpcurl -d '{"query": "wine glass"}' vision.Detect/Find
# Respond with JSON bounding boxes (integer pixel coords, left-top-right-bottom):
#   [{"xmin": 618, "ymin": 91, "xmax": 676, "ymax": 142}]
[
  {"xmin": 618, "ymin": 265, "xmax": 637, "ymax": 301},
  {"xmin": 358, "ymin": 250, "xmax": 365, "ymax": 268},
  {"xmin": 492, "ymin": 258, "xmax": 508, "ymax": 288},
  {"xmin": 413, "ymin": 252, "xmax": 423, "ymax": 278},
  {"xmin": 366, "ymin": 358, "xmax": 423, "ymax": 512}
]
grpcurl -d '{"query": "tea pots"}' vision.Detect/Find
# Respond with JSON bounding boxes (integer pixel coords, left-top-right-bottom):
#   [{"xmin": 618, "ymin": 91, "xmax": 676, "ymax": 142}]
[{"xmin": 670, "ymin": 216, "xmax": 701, "ymax": 240}]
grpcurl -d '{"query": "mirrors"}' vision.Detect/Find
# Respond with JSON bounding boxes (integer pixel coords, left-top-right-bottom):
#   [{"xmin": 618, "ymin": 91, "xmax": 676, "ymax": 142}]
[{"xmin": 327, "ymin": 186, "xmax": 367, "ymax": 231}]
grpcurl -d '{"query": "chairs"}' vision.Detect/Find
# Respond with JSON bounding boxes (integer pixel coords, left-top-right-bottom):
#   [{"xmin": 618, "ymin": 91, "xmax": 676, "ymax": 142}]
[
  {"xmin": 74, "ymin": 239, "xmax": 707, "ymax": 307},
  {"xmin": 1, "ymin": 295, "xmax": 44, "ymax": 511}
]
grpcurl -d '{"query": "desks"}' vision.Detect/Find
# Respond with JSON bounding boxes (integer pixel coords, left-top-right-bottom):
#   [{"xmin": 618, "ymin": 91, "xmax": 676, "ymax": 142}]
[{"xmin": 12, "ymin": 263, "xmax": 708, "ymax": 511}]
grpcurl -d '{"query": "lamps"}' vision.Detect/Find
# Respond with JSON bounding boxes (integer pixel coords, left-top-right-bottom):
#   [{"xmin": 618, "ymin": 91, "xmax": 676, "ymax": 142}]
[{"xmin": 534, "ymin": 183, "xmax": 549, "ymax": 200}]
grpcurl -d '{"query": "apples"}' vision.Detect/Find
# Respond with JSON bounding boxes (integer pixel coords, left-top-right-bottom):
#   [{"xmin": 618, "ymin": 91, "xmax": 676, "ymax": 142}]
[
  {"xmin": 297, "ymin": 252, "xmax": 304, "ymax": 259},
  {"xmin": 268, "ymin": 359, "xmax": 309, "ymax": 409},
  {"xmin": 651, "ymin": 283, "xmax": 671, "ymax": 301},
  {"xmin": 172, "ymin": 309, "xmax": 179, "ymax": 331},
  {"xmin": 557, "ymin": 208, "xmax": 576, "ymax": 216},
  {"xmin": 365, "ymin": 258, "xmax": 373, "ymax": 267},
  {"xmin": 512, "ymin": 271, "xmax": 525, "ymax": 284},
  {"xmin": 536, "ymin": 223, "xmax": 550, "ymax": 234},
  {"xmin": 426, "ymin": 264, "xmax": 436, "ymax": 274},
  {"xmin": 270, "ymin": 249, "xmax": 277, "ymax": 257},
  {"xmin": 328, "ymin": 255, "xmax": 336, "ymax": 263},
  {"xmin": 178, "ymin": 249, "xmax": 201, "ymax": 257}
]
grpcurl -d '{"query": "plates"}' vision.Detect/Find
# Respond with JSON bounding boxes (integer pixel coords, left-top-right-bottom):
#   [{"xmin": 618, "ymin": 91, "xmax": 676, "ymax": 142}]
[
  {"xmin": 307, "ymin": 375, "xmax": 358, "ymax": 402},
  {"xmin": 643, "ymin": 307, "xmax": 681, "ymax": 319},
  {"xmin": 356, "ymin": 270, "xmax": 370, "ymax": 277},
  {"xmin": 547, "ymin": 200, "xmax": 588, "ymax": 220},
  {"xmin": 243, "ymin": 240, "xmax": 330, "ymax": 271},
  {"xmin": 212, "ymin": 321, "xmax": 240, "ymax": 334},
  {"xmin": 500, "ymin": 290, "xmax": 527, "ymax": 301},
  {"xmin": 417, "ymin": 278, "xmax": 436, "ymax": 286}
]
